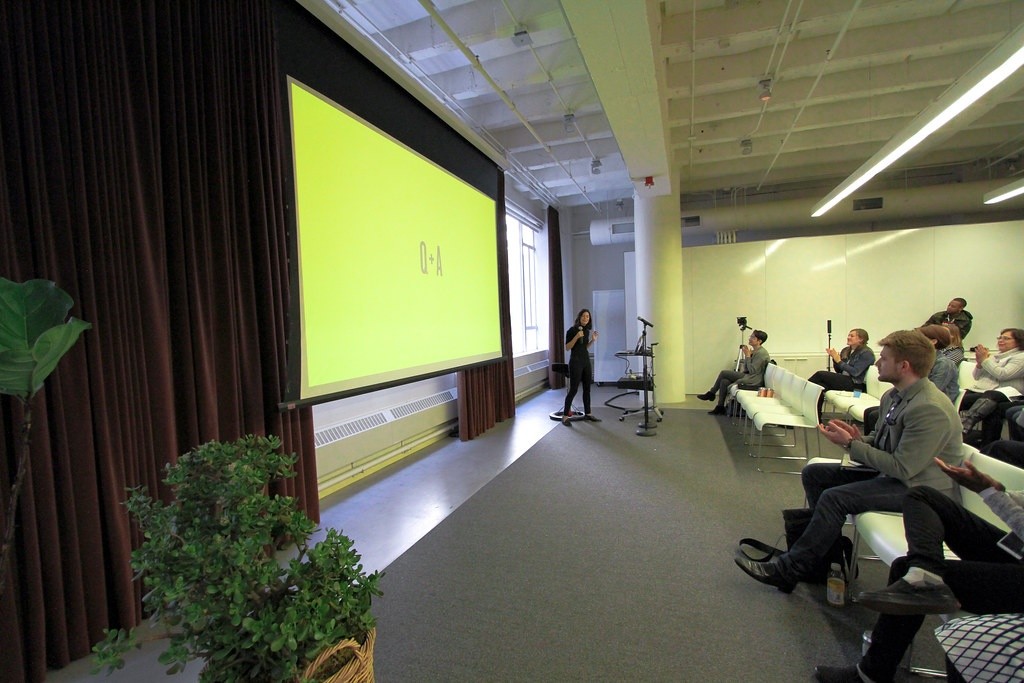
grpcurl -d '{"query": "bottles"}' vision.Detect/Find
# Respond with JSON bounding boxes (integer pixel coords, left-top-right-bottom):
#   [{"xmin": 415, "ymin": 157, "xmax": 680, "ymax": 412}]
[{"xmin": 827, "ymin": 563, "xmax": 846, "ymax": 608}]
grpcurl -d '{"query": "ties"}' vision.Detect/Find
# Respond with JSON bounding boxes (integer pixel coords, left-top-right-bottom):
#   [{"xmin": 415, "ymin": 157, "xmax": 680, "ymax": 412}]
[{"xmin": 882, "ymin": 401, "xmax": 897, "ymax": 427}]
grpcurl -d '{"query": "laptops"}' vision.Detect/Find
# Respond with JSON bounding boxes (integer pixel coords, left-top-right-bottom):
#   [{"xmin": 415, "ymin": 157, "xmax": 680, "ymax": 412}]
[{"xmin": 616, "ymin": 337, "xmax": 643, "ymax": 354}]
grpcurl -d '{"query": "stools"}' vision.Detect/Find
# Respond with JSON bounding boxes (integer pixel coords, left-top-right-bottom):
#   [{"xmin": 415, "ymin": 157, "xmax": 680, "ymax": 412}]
[{"xmin": 550, "ymin": 362, "xmax": 585, "ymax": 420}]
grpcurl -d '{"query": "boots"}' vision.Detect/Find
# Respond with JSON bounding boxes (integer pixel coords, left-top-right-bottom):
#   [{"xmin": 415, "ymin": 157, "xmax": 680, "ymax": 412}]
[{"xmin": 960, "ymin": 398, "xmax": 998, "ymax": 434}]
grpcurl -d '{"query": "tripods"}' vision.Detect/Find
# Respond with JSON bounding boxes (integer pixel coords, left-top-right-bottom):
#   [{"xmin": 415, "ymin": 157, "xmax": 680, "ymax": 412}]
[{"xmin": 734, "ymin": 324, "xmax": 752, "ymax": 371}]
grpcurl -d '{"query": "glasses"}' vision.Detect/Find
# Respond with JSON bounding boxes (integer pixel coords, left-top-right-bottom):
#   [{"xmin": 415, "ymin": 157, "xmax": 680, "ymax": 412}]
[{"xmin": 997, "ymin": 336, "xmax": 1015, "ymax": 342}]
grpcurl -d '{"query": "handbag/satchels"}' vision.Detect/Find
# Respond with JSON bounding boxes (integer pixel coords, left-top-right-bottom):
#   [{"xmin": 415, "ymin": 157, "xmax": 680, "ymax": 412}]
[{"xmin": 736, "ymin": 507, "xmax": 858, "ymax": 587}]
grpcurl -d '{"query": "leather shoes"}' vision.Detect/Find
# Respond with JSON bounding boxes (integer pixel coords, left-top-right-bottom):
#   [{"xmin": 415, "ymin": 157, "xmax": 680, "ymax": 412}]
[
  {"xmin": 853, "ymin": 579, "xmax": 960, "ymax": 615},
  {"xmin": 814, "ymin": 663, "xmax": 866, "ymax": 683},
  {"xmin": 733, "ymin": 553, "xmax": 799, "ymax": 593}
]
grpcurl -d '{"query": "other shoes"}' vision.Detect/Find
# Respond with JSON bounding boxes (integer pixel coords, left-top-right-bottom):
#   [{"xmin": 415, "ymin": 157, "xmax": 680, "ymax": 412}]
[
  {"xmin": 708, "ymin": 407, "xmax": 726, "ymax": 415},
  {"xmin": 562, "ymin": 416, "xmax": 572, "ymax": 427},
  {"xmin": 697, "ymin": 391, "xmax": 716, "ymax": 402},
  {"xmin": 584, "ymin": 414, "xmax": 601, "ymax": 422}
]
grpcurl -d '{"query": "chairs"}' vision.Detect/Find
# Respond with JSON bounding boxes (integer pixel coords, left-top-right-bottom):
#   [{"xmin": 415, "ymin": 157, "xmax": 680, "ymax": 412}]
[{"xmin": 728, "ymin": 361, "xmax": 1024, "ymax": 683}]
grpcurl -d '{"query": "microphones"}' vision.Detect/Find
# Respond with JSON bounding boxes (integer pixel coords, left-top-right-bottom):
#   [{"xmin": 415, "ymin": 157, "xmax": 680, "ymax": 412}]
[{"xmin": 638, "ymin": 316, "xmax": 654, "ymax": 328}]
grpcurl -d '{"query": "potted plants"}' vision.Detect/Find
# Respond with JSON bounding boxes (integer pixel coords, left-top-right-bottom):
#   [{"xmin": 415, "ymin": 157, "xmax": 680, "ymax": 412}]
[{"xmin": 90, "ymin": 435, "xmax": 387, "ymax": 683}]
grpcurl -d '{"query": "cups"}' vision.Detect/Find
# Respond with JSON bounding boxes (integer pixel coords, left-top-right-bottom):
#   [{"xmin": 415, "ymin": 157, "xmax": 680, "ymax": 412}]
[
  {"xmin": 758, "ymin": 386, "xmax": 774, "ymax": 397},
  {"xmin": 854, "ymin": 389, "xmax": 862, "ymax": 398},
  {"xmin": 862, "ymin": 630, "xmax": 873, "ymax": 655}
]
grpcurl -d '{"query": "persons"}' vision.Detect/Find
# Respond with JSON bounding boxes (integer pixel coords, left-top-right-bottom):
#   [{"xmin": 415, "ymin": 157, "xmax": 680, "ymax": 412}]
[
  {"xmin": 914, "ymin": 325, "xmax": 964, "ymax": 402},
  {"xmin": 562, "ymin": 309, "xmax": 599, "ymax": 426},
  {"xmin": 735, "ymin": 330, "xmax": 964, "ymax": 593},
  {"xmin": 697, "ymin": 329, "xmax": 770, "ymax": 415},
  {"xmin": 959, "ymin": 328, "xmax": 1024, "ymax": 468},
  {"xmin": 815, "ymin": 456, "xmax": 1024, "ymax": 683},
  {"xmin": 778, "ymin": 329, "xmax": 875, "ymax": 429},
  {"xmin": 922, "ymin": 298, "xmax": 974, "ymax": 339}
]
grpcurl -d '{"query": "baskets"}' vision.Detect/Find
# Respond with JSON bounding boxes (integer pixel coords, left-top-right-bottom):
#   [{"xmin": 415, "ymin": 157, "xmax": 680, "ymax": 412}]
[{"xmin": 303, "ymin": 620, "xmax": 378, "ymax": 683}]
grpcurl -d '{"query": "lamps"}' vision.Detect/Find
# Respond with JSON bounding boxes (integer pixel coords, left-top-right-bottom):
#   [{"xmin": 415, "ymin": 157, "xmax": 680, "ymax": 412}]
[
  {"xmin": 758, "ymin": 77, "xmax": 771, "ymax": 100},
  {"xmin": 592, "ymin": 160, "xmax": 602, "ymax": 175},
  {"xmin": 616, "ymin": 199, "xmax": 624, "ymax": 212},
  {"xmin": 564, "ymin": 114, "xmax": 576, "ymax": 132},
  {"xmin": 512, "ymin": 26, "xmax": 533, "ymax": 47},
  {"xmin": 809, "ymin": 22, "xmax": 1024, "ymax": 219},
  {"xmin": 741, "ymin": 140, "xmax": 753, "ymax": 155}
]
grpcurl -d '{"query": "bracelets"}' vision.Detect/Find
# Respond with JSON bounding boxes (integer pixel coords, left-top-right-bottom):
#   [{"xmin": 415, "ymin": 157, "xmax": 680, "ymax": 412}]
[{"xmin": 844, "ymin": 438, "xmax": 856, "ymax": 451}]
